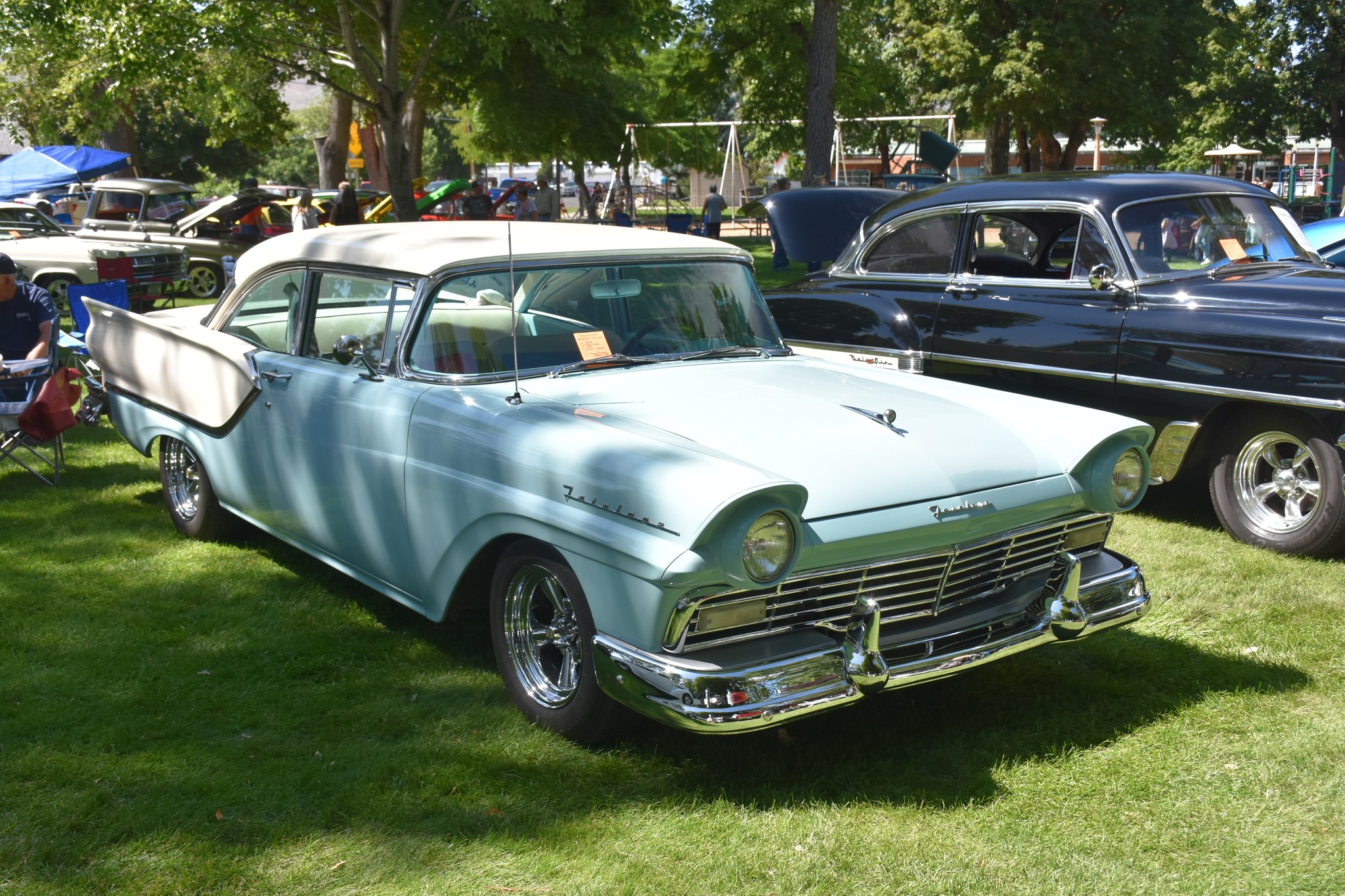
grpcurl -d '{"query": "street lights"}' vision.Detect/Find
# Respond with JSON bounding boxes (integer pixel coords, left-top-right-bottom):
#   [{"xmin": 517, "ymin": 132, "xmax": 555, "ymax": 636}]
[
  {"xmin": 1090, "ymin": 117, "xmax": 1108, "ymax": 173},
  {"xmin": 1312, "ymin": 140, "xmax": 1320, "ymax": 194},
  {"xmin": 1287, "ymin": 142, "xmax": 1298, "ymax": 204}
]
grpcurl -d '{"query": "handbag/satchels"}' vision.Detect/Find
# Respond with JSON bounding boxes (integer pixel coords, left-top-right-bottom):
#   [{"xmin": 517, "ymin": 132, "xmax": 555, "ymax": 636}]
[
  {"xmin": 329, "ymin": 205, "xmax": 338, "ymax": 225},
  {"xmin": 17, "ymin": 366, "xmax": 90, "ymax": 443}
]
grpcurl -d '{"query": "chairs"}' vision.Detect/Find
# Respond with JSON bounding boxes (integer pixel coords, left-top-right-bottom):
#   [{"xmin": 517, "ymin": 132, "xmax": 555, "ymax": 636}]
[
  {"xmin": 614, "ymin": 213, "xmax": 703, "ymax": 236},
  {"xmin": 0, "ymin": 314, "xmax": 65, "ymax": 486},
  {"xmin": 67, "ymin": 278, "xmax": 129, "ymax": 408},
  {"xmin": 96, "ymin": 256, "xmax": 175, "ymax": 313}
]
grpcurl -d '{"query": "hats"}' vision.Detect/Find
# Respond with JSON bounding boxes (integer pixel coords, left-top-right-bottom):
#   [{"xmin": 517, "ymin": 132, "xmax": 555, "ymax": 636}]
[
  {"xmin": 537, "ymin": 174, "xmax": 548, "ymax": 183},
  {"xmin": 471, "ymin": 180, "xmax": 482, "ymax": 189},
  {"xmin": 0, "ymin": 252, "xmax": 17, "ymax": 275}
]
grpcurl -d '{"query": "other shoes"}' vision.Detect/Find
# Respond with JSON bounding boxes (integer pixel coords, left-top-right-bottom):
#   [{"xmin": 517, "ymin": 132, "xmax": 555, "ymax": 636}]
[{"xmin": 1200, "ymin": 256, "xmax": 1214, "ymax": 265}]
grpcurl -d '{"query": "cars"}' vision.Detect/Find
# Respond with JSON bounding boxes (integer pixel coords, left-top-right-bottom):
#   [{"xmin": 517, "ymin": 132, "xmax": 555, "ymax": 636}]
[
  {"xmin": 84, "ymin": 221, "xmax": 1150, "ymax": 746},
  {"xmin": 1191, "ymin": 215, "xmax": 1344, "ymax": 273},
  {"xmin": 0, "ymin": 201, "xmax": 190, "ymax": 315},
  {"xmin": 731, "ymin": 174, "xmax": 1345, "ymax": 561},
  {"xmin": 14, "ymin": 178, "xmax": 653, "ymax": 298}
]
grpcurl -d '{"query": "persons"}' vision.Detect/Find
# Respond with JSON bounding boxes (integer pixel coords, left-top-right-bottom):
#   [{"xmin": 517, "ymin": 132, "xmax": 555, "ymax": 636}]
[
  {"xmin": 593, "ymin": 182, "xmax": 603, "ymax": 208},
  {"xmin": 1244, "ymin": 177, "xmax": 1273, "ymax": 244},
  {"xmin": 462, "ymin": 181, "xmax": 497, "ymax": 221},
  {"xmin": 805, "ymin": 168, "xmax": 834, "ymax": 274},
  {"xmin": 238, "ymin": 178, "xmax": 261, "ymax": 242},
  {"xmin": 329, "ymin": 182, "xmax": 360, "ymax": 226},
  {"xmin": 1137, "ymin": 210, "xmax": 1217, "ymax": 265},
  {"xmin": 512, "ymin": 175, "xmax": 557, "ymax": 222},
  {"xmin": 0, "ymin": 253, "xmax": 56, "ymax": 402},
  {"xmin": 291, "ymin": 190, "xmax": 319, "ymax": 232},
  {"xmin": 770, "ymin": 176, "xmax": 792, "ymax": 269},
  {"xmin": 700, "ymin": 184, "xmax": 727, "ymax": 240},
  {"xmin": 36, "ymin": 201, "xmax": 60, "ymax": 226}
]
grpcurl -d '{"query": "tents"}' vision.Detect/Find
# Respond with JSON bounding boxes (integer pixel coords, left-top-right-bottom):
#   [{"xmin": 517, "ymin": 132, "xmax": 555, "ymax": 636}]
[
  {"xmin": 0, "ymin": 145, "xmax": 132, "ymax": 221},
  {"xmin": 1204, "ymin": 144, "xmax": 1263, "ymax": 181}
]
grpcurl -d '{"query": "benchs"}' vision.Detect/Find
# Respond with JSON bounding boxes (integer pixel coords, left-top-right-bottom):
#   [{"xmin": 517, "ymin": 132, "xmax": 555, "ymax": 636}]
[
  {"xmin": 865, "ymin": 253, "xmax": 1032, "ymax": 278},
  {"xmin": 237, "ymin": 308, "xmax": 531, "ymax": 374}
]
